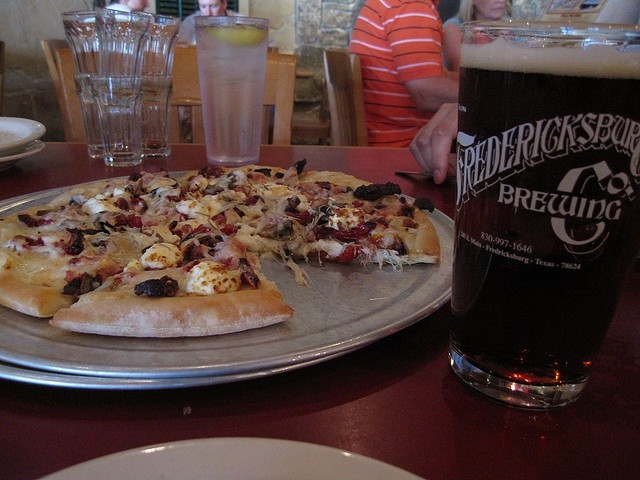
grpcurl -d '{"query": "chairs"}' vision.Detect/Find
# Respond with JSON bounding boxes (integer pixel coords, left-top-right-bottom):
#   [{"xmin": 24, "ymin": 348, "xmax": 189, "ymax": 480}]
[
  {"xmin": 321, "ymin": 49, "xmax": 369, "ymax": 145},
  {"xmin": 40, "ymin": 37, "xmax": 86, "ymax": 142},
  {"xmin": 75, "ymin": 52, "xmax": 182, "ymax": 145},
  {"xmin": 172, "ymin": 42, "xmax": 297, "ymax": 145}
]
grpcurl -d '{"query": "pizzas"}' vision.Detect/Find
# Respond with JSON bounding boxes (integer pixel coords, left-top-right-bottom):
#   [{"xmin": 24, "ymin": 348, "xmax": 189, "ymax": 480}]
[{"xmin": 0, "ymin": 158, "xmax": 439, "ymax": 338}]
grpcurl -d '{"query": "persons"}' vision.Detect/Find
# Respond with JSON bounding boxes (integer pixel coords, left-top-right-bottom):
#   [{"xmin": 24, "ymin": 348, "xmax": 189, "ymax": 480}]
[
  {"xmin": 84, "ymin": 0, "xmax": 152, "ymax": 39},
  {"xmin": 176, "ymin": 1, "xmax": 247, "ymax": 45},
  {"xmin": 409, "ymin": 0, "xmax": 639, "ymax": 185},
  {"xmin": 347, "ymin": 0, "xmax": 460, "ymax": 149},
  {"xmin": 440, "ymin": 0, "xmax": 514, "ymax": 73}
]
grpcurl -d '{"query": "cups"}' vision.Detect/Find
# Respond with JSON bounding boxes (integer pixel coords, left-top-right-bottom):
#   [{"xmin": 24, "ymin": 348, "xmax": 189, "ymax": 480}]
[
  {"xmin": 128, "ymin": 10, "xmax": 180, "ymax": 88},
  {"xmin": 138, "ymin": 75, "xmax": 172, "ymax": 159},
  {"xmin": 95, "ymin": 7, "xmax": 150, "ymax": 90},
  {"xmin": 61, "ymin": 10, "xmax": 100, "ymax": 75},
  {"xmin": 192, "ymin": 15, "xmax": 269, "ymax": 167},
  {"xmin": 445, "ymin": 19, "xmax": 640, "ymax": 415},
  {"xmin": 91, "ymin": 74, "xmax": 144, "ymax": 168},
  {"xmin": 73, "ymin": 72, "xmax": 108, "ymax": 160}
]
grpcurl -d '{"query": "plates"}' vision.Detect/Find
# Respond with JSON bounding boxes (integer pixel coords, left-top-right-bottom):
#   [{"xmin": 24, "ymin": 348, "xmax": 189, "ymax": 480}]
[
  {"xmin": 2, "ymin": 346, "xmax": 356, "ymax": 390},
  {"xmin": 0, "ymin": 139, "xmax": 46, "ymax": 172},
  {"xmin": 0, "ymin": 170, "xmax": 458, "ymax": 376},
  {"xmin": 30, "ymin": 437, "xmax": 432, "ymax": 480}
]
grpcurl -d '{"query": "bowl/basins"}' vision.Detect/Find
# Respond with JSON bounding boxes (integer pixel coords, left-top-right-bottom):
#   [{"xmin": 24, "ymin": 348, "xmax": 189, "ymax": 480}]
[{"xmin": 0, "ymin": 116, "xmax": 47, "ymax": 157}]
